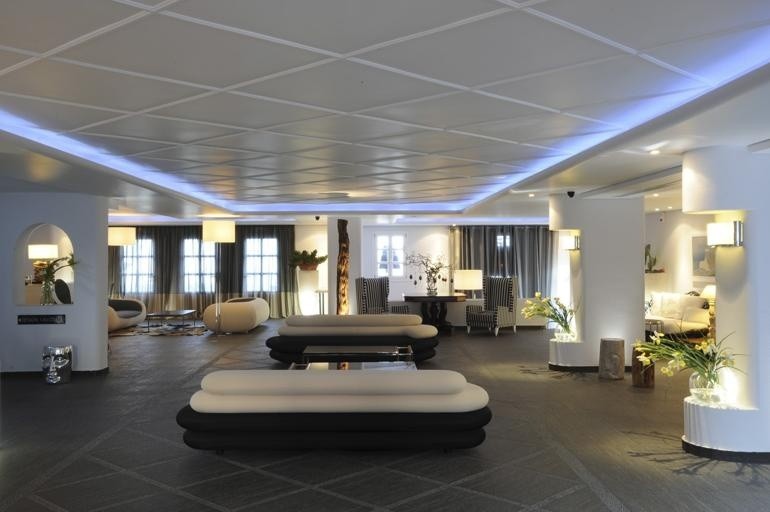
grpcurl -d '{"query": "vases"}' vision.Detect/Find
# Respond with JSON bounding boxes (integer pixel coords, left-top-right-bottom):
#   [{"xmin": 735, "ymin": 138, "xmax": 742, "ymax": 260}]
[
  {"xmin": 426, "ymin": 277, "xmax": 438, "ymax": 295},
  {"xmin": 555, "ymin": 322, "xmax": 575, "ymax": 340},
  {"xmin": 689, "ymin": 369, "xmax": 723, "ymax": 403},
  {"xmin": 38, "ymin": 282, "xmax": 58, "ymax": 305}
]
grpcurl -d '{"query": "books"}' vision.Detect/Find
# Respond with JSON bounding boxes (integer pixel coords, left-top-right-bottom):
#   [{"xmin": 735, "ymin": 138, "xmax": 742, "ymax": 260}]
[{"xmin": 307, "ymin": 361, "xmax": 329, "ymax": 370}]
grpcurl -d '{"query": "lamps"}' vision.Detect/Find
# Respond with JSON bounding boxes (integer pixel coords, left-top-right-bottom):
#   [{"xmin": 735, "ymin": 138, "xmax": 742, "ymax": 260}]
[
  {"xmin": 107, "ymin": 227, "xmax": 136, "ymax": 247},
  {"xmin": 701, "ymin": 285, "xmax": 716, "ymax": 315},
  {"xmin": 706, "ymin": 220, "xmax": 744, "ymax": 247},
  {"xmin": 558, "ymin": 235, "xmax": 579, "ymax": 251},
  {"xmin": 453, "ymin": 269, "xmax": 483, "ymax": 299},
  {"xmin": 201, "ymin": 219, "xmax": 235, "ymax": 337}
]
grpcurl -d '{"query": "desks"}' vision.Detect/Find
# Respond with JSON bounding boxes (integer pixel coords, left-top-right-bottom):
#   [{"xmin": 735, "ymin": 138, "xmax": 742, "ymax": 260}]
[
  {"xmin": 645, "ymin": 319, "xmax": 664, "ymax": 340},
  {"xmin": 404, "ymin": 294, "xmax": 467, "ymax": 334}
]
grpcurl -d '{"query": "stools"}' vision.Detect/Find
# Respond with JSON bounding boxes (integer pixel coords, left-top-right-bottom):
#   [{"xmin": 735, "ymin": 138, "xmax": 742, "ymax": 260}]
[
  {"xmin": 630, "ymin": 344, "xmax": 656, "ymax": 388},
  {"xmin": 597, "ymin": 337, "xmax": 625, "ymax": 379}
]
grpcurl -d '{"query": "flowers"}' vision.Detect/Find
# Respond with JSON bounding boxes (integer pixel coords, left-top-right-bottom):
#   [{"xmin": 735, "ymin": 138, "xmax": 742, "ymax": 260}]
[
  {"xmin": 28, "ymin": 254, "xmax": 77, "ymax": 300},
  {"xmin": 632, "ymin": 317, "xmax": 747, "ymax": 394},
  {"xmin": 406, "ymin": 254, "xmax": 454, "ymax": 287},
  {"xmin": 522, "ymin": 293, "xmax": 582, "ymax": 332}
]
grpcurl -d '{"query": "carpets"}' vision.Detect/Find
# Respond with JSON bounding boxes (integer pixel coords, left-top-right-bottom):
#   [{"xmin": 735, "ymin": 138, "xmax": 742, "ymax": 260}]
[{"xmin": 112, "ymin": 322, "xmax": 207, "ymax": 338}]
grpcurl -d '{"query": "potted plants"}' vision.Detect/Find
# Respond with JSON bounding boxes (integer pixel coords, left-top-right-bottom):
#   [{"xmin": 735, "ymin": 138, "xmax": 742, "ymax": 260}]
[{"xmin": 287, "ymin": 250, "xmax": 329, "ymax": 271}]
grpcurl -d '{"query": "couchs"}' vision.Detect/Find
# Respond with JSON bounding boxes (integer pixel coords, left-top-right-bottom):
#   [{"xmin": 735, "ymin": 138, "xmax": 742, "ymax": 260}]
[
  {"xmin": 107, "ymin": 297, "xmax": 147, "ymax": 333},
  {"xmin": 203, "ymin": 296, "xmax": 271, "ymax": 334},
  {"xmin": 264, "ymin": 315, "xmax": 438, "ymax": 365},
  {"xmin": 466, "ymin": 274, "xmax": 520, "ymax": 334},
  {"xmin": 646, "ymin": 293, "xmax": 709, "ymax": 338},
  {"xmin": 175, "ymin": 368, "xmax": 493, "ymax": 456},
  {"xmin": 356, "ymin": 277, "xmax": 409, "ymax": 316}
]
